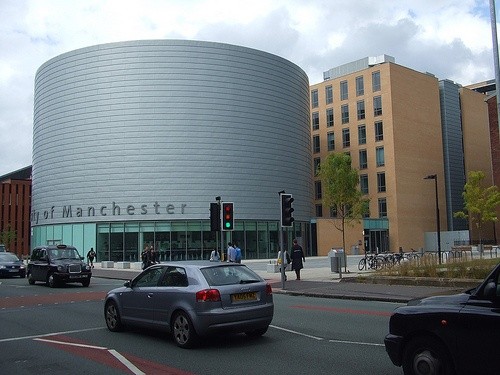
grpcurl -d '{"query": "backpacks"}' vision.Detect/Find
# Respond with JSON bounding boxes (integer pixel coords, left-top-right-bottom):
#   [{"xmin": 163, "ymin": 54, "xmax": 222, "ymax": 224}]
[
  {"xmin": 294, "ymin": 250, "xmax": 302, "ymax": 258},
  {"xmin": 90, "ymin": 252, "xmax": 95, "ymax": 257}
]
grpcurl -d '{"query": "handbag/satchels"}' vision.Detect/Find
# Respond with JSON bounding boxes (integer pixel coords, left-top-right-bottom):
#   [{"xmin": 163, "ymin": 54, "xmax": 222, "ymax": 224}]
[
  {"xmin": 286, "ymin": 264, "xmax": 292, "ymax": 271},
  {"xmin": 286, "ymin": 252, "xmax": 290, "ymax": 264},
  {"xmin": 277, "ymin": 258, "xmax": 282, "ymax": 263},
  {"xmin": 275, "ymin": 263, "xmax": 280, "ymax": 273}
]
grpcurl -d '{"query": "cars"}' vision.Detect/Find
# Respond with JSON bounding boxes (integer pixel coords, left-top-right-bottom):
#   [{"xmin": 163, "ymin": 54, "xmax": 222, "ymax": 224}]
[
  {"xmin": 0, "ymin": 251, "xmax": 26, "ymax": 279},
  {"xmin": 383, "ymin": 261, "xmax": 500, "ymax": 375},
  {"xmin": 103, "ymin": 259, "xmax": 275, "ymax": 349}
]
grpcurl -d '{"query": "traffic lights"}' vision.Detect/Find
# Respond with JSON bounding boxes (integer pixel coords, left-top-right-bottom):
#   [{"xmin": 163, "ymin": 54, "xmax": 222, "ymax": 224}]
[
  {"xmin": 223, "ymin": 202, "xmax": 233, "ymax": 230},
  {"xmin": 282, "ymin": 194, "xmax": 295, "ymax": 227}
]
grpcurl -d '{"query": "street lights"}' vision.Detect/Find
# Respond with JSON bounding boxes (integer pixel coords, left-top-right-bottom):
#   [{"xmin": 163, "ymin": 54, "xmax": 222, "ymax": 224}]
[{"xmin": 423, "ymin": 174, "xmax": 442, "ymax": 264}]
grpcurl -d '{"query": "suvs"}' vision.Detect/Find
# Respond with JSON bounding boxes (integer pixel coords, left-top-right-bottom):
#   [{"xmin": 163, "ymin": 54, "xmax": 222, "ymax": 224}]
[{"xmin": 26, "ymin": 244, "xmax": 92, "ymax": 288}]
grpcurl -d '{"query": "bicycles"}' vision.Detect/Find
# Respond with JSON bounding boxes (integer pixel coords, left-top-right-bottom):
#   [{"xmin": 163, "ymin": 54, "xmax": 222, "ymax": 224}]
[{"xmin": 357, "ymin": 248, "xmax": 425, "ymax": 272}]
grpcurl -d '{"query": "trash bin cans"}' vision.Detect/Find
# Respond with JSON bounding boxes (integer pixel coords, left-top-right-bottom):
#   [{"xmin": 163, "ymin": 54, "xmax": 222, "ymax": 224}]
[
  {"xmin": 329, "ymin": 247, "xmax": 347, "ymax": 273},
  {"xmin": 352, "ymin": 246, "xmax": 360, "ymax": 255}
]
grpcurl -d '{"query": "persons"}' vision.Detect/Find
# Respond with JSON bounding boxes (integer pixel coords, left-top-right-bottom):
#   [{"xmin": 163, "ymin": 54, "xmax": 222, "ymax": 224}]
[
  {"xmin": 227, "ymin": 242, "xmax": 237, "ymax": 263},
  {"xmin": 277, "ymin": 245, "xmax": 291, "ymax": 281},
  {"xmin": 141, "ymin": 245, "xmax": 161, "ymax": 271},
  {"xmin": 234, "ymin": 243, "xmax": 242, "ymax": 264},
  {"xmin": 211, "ymin": 247, "xmax": 220, "ymax": 262},
  {"xmin": 87, "ymin": 248, "xmax": 97, "ymax": 268},
  {"xmin": 290, "ymin": 240, "xmax": 305, "ymax": 280}
]
